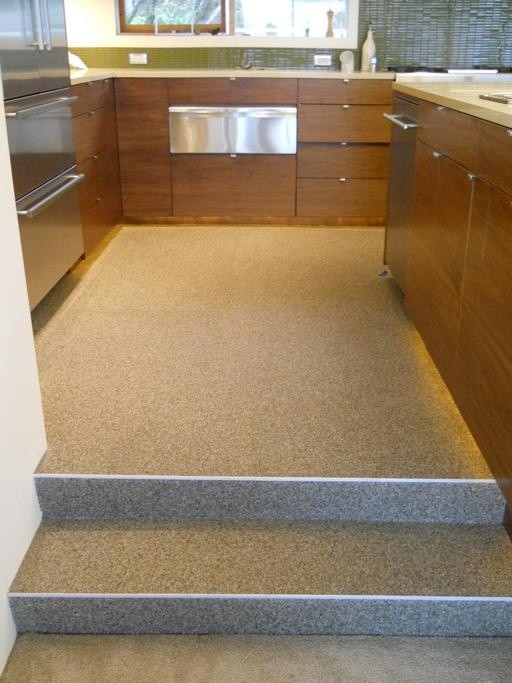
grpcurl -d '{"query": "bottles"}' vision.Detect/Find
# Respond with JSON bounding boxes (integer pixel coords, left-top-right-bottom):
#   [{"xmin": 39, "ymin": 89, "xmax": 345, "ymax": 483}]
[{"xmin": 361, "ymin": 30, "xmax": 376, "ymax": 72}]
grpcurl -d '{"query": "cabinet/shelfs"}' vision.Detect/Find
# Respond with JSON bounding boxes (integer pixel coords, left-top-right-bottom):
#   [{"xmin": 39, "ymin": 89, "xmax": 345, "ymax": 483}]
[
  {"xmin": 69, "ymin": 77, "xmax": 122, "ymax": 262},
  {"xmin": 168, "ymin": 77, "xmax": 392, "ymax": 227},
  {"xmin": 384, "ymin": 91, "xmax": 512, "ymax": 507}
]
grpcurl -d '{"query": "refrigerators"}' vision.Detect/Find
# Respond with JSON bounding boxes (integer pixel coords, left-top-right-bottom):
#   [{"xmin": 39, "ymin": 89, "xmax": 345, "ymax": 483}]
[{"xmin": 0, "ymin": 0, "xmax": 86, "ymax": 316}]
